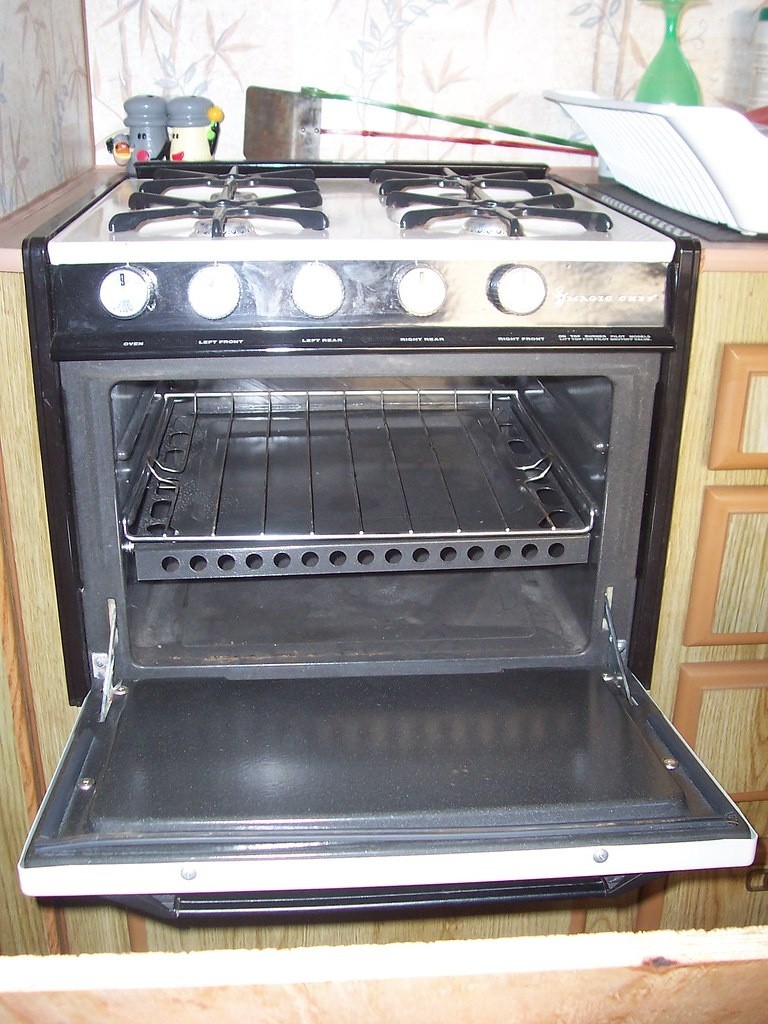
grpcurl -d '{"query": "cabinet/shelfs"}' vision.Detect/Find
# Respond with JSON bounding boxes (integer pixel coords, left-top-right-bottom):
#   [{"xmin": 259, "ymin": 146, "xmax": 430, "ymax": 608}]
[{"xmin": 644, "ymin": 269, "xmax": 768, "ymax": 933}]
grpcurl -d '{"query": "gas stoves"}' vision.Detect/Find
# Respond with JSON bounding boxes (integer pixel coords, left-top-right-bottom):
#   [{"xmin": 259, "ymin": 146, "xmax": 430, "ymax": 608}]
[{"xmin": 109, "ymin": 168, "xmax": 612, "ymax": 237}]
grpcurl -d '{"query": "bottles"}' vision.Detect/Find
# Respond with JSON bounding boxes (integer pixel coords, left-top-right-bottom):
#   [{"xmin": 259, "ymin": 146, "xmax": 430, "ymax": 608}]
[
  {"xmin": 746, "ymin": 7, "xmax": 768, "ymax": 132},
  {"xmin": 123, "ymin": 95, "xmax": 217, "ymax": 175}
]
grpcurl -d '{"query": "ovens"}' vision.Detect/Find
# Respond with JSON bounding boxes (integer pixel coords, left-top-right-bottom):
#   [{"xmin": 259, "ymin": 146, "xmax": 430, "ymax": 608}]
[{"xmin": 19, "ymin": 346, "xmax": 761, "ymax": 919}]
